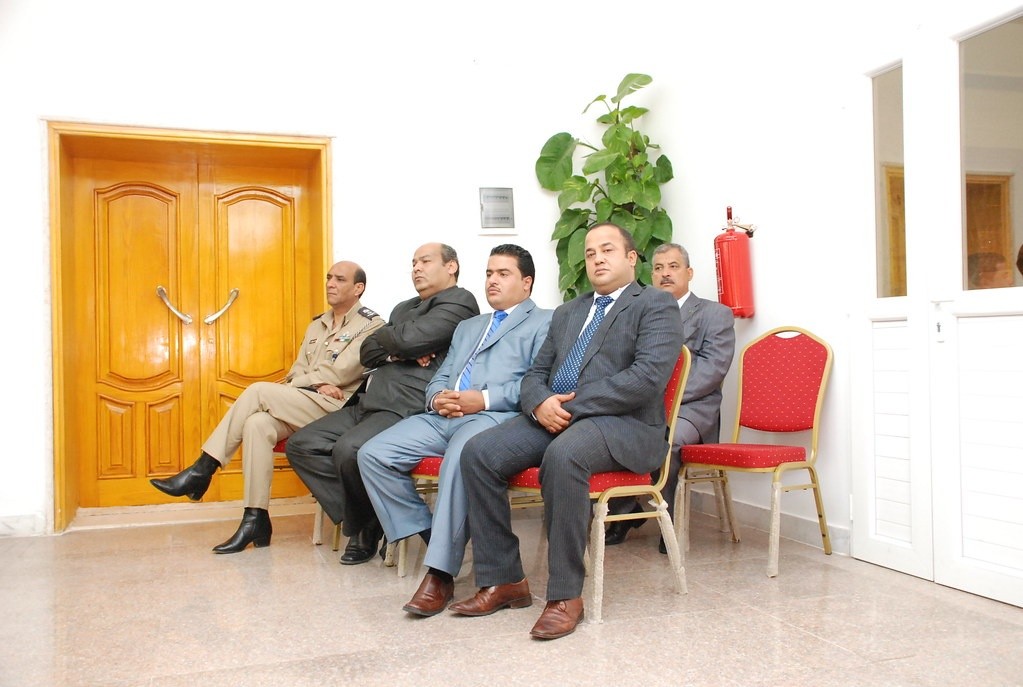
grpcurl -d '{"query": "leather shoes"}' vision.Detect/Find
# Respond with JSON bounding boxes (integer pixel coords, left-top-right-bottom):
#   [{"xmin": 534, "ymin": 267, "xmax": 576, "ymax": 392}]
[
  {"xmin": 449, "ymin": 578, "xmax": 533, "ymax": 617},
  {"xmin": 404, "ymin": 573, "xmax": 456, "ymax": 617},
  {"xmin": 529, "ymin": 599, "xmax": 584, "ymax": 638},
  {"xmin": 339, "ymin": 520, "xmax": 385, "ymax": 565},
  {"xmin": 659, "ymin": 537, "xmax": 671, "ymax": 555},
  {"xmin": 605, "ymin": 501, "xmax": 648, "ymax": 546}
]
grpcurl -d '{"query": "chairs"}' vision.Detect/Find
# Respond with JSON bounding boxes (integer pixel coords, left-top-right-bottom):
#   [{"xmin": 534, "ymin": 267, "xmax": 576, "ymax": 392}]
[
  {"xmin": 674, "ymin": 326, "xmax": 835, "ymax": 580},
  {"xmin": 272, "ymin": 436, "xmax": 444, "ymax": 578},
  {"xmin": 508, "ymin": 343, "xmax": 692, "ymax": 626}
]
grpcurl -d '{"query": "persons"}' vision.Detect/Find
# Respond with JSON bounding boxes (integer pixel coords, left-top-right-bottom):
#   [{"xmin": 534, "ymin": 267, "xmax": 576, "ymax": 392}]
[
  {"xmin": 601, "ymin": 241, "xmax": 735, "ymax": 555},
  {"xmin": 355, "ymin": 245, "xmax": 551, "ymax": 615},
  {"xmin": 152, "ymin": 260, "xmax": 390, "ymax": 553},
  {"xmin": 444, "ymin": 222, "xmax": 683, "ymax": 637},
  {"xmin": 285, "ymin": 242, "xmax": 479, "ymax": 563}
]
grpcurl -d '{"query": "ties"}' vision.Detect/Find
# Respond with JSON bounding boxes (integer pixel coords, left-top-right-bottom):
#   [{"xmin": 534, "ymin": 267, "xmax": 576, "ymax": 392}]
[
  {"xmin": 552, "ymin": 296, "xmax": 614, "ymax": 394},
  {"xmin": 460, "ymin": 311, "xmax": 508, "ymax": 392}
]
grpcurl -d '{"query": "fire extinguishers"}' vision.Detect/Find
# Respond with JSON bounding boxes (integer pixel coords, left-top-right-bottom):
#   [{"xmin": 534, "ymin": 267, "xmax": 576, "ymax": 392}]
[{"xmin": 714, "ymin": 206, "xmax": 758, "ymax": 319}]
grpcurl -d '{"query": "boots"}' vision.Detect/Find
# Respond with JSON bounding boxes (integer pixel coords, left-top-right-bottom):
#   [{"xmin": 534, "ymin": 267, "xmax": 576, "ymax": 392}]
[
  {"xmin": 150, "ymin": 453, "xmax": 219, "ymax": 500},
  {"xmin": 211, "ymin": 508, "xmax": 274, "ymax": 554}
]
